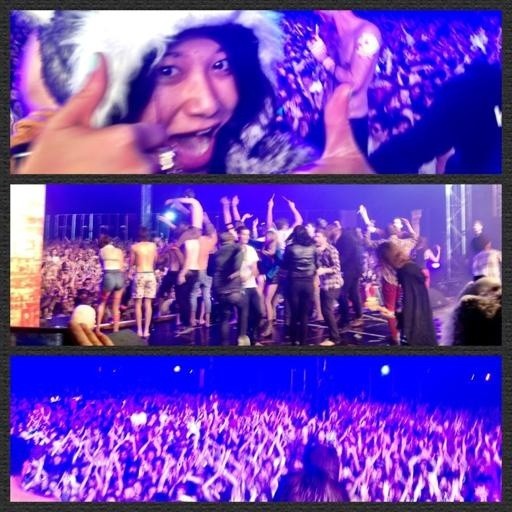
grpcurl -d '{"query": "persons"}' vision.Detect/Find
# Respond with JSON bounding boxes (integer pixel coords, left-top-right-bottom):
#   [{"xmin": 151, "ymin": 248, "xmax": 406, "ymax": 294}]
[
  {"xmin": 41, "ymin": 189, "xmax": 502, "ymax": 348},
  {"xmin": 7, "ymin": 7, "xmax": 504, "ymax": 174},
  {"xmin": 9, "ymin": 392, "xmax": 502, "ymax": 502}
]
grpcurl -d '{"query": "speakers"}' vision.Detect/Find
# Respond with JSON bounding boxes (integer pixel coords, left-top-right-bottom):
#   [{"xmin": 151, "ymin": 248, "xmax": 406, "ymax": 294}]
[{"xmin": 109, "ymin": 329, "xmax": 147, "ymax": 346}]
